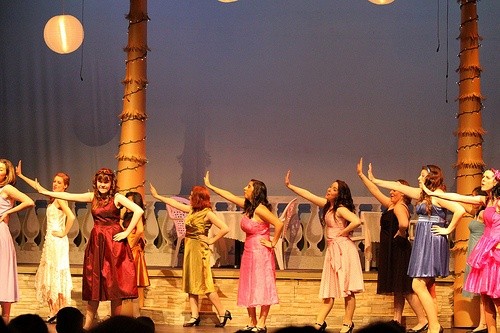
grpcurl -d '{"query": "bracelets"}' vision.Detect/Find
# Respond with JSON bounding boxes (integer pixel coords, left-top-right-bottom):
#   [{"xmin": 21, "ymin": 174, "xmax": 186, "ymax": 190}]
[
  {"xmin": 360, "ymin": 175, "xmax": 365, "ymax": 180},
  {"xmin": 359, "ymin": 173, "xmax": 363, "ymax": 177},
  {"xmin": 270, "ymin": 241, "xmax": 275, "ymax": 248}
]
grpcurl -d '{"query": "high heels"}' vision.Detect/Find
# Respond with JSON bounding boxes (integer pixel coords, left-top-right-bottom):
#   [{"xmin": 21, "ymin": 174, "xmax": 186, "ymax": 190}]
[
  {"xmin": 215, "ymin": 310, "xmax": 232, "ymax": 327},
  {"xmin": 339, "ymin": 321, "xmax": 354, "ymax": 333},
  {"xmin": 315, "ymin": 320, "xmax": 327, "ymax": 333},
  {"xmin": 44, "ymin": 314, "xmax": 57, "ymax": 323},
  {"xmin": 183, "ymin": 316, "xmax": 201, "ymax": 327}
]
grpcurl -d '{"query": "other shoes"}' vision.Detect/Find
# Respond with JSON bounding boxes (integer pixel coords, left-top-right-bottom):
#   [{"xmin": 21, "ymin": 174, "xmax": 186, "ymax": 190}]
[
  {"xmin": 408, "ymin": 322, "xmax": 428, "ymax": 333},
  {"xmin": 251, "ymin": 325, "xmax": 267, "ymax": 333},
  {"xmin": 243, "ymin": 325, "xmax": 256, "ymax": 331},
  {"xmin": 439, "ymin": 326, "xmax": 444, "ymax": 333},
  {"xmin": 466, "ymin": 327, "xmax": 488, "ymax": 333}
]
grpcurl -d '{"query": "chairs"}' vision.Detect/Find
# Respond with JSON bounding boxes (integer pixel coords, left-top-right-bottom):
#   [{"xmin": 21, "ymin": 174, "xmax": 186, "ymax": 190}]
[
  {"xmin": 166, "ymin": 197, "xmax": 190, "ymax": 268},
  {"xmin": 270, "ymin": 198, "xmax": 298, "ymax": 270}
]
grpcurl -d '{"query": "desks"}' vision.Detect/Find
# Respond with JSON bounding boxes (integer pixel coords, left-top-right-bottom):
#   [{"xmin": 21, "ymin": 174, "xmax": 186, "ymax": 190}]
[
  {"xmin": 208, "ymin": 211, "xmax": 246, "ymax": 268},
  {"xmin": 361, "ymin": 211, "xmax": 382, "ymax": 271}
]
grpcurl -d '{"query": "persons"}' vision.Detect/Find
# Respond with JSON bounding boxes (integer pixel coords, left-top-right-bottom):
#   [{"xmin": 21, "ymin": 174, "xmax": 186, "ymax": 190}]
[
  {"xmin": 149, "ymin": 183, "xmax": 233, "ymax": 328},
  {"xmin": 368, "ymin": 162, "xmax": 466, "ymax": 333},
  {"xmin": 0, "ymin": 158, "xmax": 35, "ymax": 326},
  {"xmin": 0, "ymin": 307, "xmax": 408, "ymax": 333},
  {"xmin": 459, "ymin": 186, "xmax": 490, "ymax": 333},
  {"xmin": 356, "ymin": 157, "xmax": 428, "ymax": 333},
  {"xmin": 422, "ymin": 168, "xmax": 500, "ymax": 333},
  {"xmin": 34, "ymin": 168, "xmax": 145, "ymax": 331},
  {"xmin": 120, "ymin": 192, "xmax": 151, "ymax": 317},
  {"xmin": 203, "ymin": 170, "xmax": 284, "ymax": 333},
  {"xmin": 284, "ymin": 170, "xmax": 366, "ymax": 333},
  {"xmin": 15, "ymin": 159, "xmax": 75, "ymax": 324}
]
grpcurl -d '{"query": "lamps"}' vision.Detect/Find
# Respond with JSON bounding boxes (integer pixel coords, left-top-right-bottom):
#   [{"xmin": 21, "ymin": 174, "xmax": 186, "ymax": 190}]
[{"xmin": 43, "ymin": 0, "xmax": 85, "ymax": 55}]
